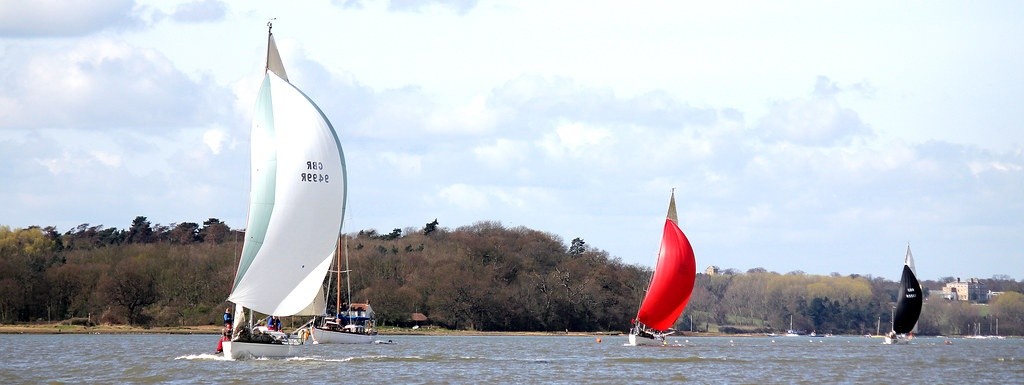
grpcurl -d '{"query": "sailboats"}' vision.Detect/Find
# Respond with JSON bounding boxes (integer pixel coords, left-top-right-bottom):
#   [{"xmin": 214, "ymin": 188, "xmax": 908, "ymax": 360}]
[
  {"xmin": 222, "ymin": 17, "xmax": 347, "ymax": 360},
  {"xmin": 884, "ymin": 242, "xmax": 923, "ymax": 345},
  {"xmin": 309, "ymin": 233, "xmax": 378, "ymax": 344},
  {"xmin": 963, "ymin": 318, "xmax": 1007, "ymax": 338},
  {"xmin": 629, "ymin": 188, "xmax": 696, "ymax": 346},
  {"xmin": 786, "ymin": 315, "xmax": 799, "ymax": 336}
]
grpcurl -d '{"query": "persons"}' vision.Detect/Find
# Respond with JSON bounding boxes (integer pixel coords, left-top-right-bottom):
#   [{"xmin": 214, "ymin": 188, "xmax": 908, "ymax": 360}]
[
  {"xmin": 268, "ymin": 316, "xmax": 281, "ymax": 332},
  {"xmin": 659, "ymin": 331, "xmax": 665, "ymax": 340},
  {"xmin": 300, "ymin": 330, "xmax": 304, "ymax": 345},
  {"xmin": 223, "ymin": 308, "xmax": 233, "ymax": 331},
  {"xmin": 306, "ymin": 329, "xmax": 310, "ymax": 340},
  {"xmin": 368, "ymin": 319, "xmax": 373, "ymax": 329},
  {"xmin": 367, "ymin": 299, "xmax": 370, "ymax": 306},
  {"xmin": 632, "ymin": 317, "xmax": 636, "ymax": 334}
]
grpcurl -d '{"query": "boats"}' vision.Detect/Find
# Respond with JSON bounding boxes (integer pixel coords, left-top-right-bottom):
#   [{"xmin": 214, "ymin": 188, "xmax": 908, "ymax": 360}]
[
  {"xmin": 811, "ymin": 331, "xmax": 816, "ymax": 336},
  {"xmin": 825, "ymin": 332, "xmax": 832, "ymax": 337},
  {"xmin": 767, "ymin": 333, "xmax": 779, "ymax": 337},
  {"xmin": 375, "ymin": 340, "xmax": 398, "ymax": 345},
  {"xmin": 944, "ymin": 341, "xmax": 953, "ymax": 345}
]
